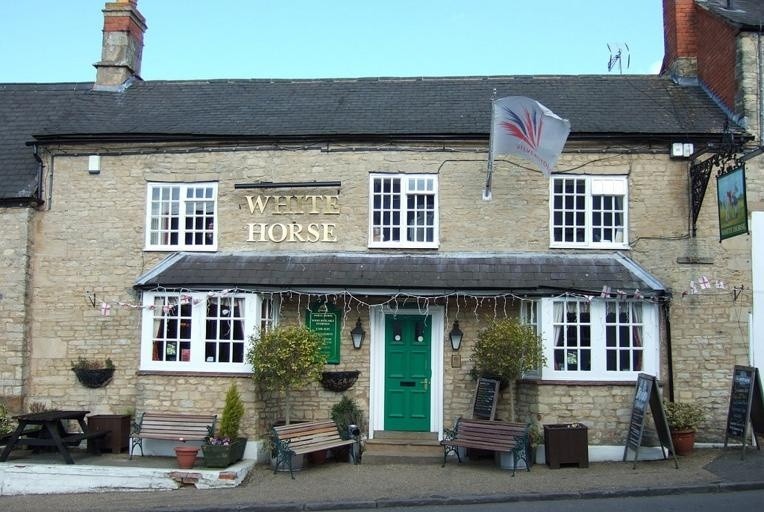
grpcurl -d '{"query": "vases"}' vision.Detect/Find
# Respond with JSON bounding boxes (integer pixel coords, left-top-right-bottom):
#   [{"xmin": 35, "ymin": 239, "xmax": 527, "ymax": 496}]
[{"xmin": 173, "ymin": 446, "xmax": 198, "ymax": 469}]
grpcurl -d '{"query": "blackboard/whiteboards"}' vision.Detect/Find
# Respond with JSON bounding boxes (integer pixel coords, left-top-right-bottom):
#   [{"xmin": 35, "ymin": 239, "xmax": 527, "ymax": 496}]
[
  {"xmin": 725, "ymin": 364, "xmax": 752, "ymax": 442},
  {"xmin": 751, "ymin": 367, "xmax": 764, "ymax": 436},
  {"xmin": 474, "ymin": 378, "xmax": 500, "ymax": 418},
  {"xmin": 626, "ymin": 373, "xmax": 651, "ymax": 451},
  {"xmin": 649, "ymin": 377, "xmax": 674, "ymax": 449}
]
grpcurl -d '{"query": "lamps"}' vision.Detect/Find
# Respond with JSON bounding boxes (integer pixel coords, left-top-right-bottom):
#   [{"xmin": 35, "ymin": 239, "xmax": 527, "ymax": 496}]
[
  {"xmin": 348, "ymin": 316, "xmax": 367, "ymax": 350},
  {"xmin": 449, "ymin": 319, "xmax": 463, "ymax": 351}
]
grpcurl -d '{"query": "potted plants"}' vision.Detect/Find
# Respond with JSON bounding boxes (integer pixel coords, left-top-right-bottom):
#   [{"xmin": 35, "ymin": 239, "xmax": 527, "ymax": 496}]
[
  {"xmin": 470, "ymin": 316, "xmax": 547, "ymax": 471},
  {"xmin": 202, "ymin": 382, "xmax": 247, "ymax": 467},
  {"xmin": 247, "ymin": 323, "xmax": 328, "ymax": 472}
]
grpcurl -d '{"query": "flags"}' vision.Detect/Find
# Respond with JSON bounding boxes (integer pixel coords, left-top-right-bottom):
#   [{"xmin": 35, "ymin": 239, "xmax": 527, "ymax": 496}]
[{"xmin": 491, "ymin": 96, "xmax": 571, "ymax": 177}]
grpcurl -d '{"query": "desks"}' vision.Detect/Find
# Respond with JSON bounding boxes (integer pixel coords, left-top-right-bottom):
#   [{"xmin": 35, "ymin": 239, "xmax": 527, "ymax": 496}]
[{"xmin": 0, "ymin": 411, "xmax": 101, "ymax": 464}]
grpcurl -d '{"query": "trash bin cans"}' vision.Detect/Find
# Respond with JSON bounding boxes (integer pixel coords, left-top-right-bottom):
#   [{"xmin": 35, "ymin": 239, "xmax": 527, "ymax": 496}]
[{"xmin": 347, "ymin": 424, "xmax": 360, "ymax": 466}]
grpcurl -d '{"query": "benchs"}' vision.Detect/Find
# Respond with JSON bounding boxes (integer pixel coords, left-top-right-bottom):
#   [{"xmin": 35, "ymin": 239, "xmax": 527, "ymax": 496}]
[
  {"xmin": 128, "ymin": 411, "xmax": 215, "ymax": 461},
  {"xmin": 272, "ymin": 419, "xmax": 358, "ymax": 479},
  {"xmin": 0, "ymin": 429, "xmax": 39, "ymax": 442},
  {"xmin": 440, "ymin": 415, "xmax": 532, "ymax": 477},
  {"xmin": 62, "ymin": 429, "xmax": 114, "ymax": 444}
]
